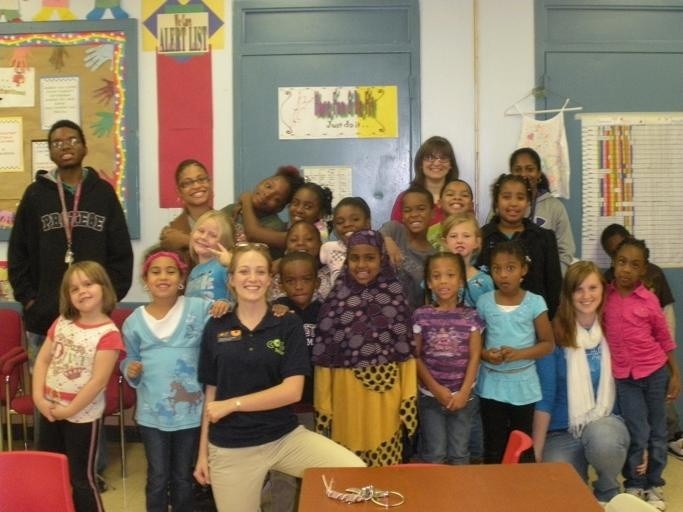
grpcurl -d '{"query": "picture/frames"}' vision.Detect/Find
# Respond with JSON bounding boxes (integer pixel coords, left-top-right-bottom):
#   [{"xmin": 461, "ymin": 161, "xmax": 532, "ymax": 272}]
[{"xmin": 0, "ymin": 18, "xmax": 140, "ymax": 240}]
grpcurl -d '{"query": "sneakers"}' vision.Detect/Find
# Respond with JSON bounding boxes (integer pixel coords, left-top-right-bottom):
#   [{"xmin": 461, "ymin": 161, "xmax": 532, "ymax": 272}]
[
  {"xmin": 97, "ymin": 470, "xmax": 108, "ymax": 493},
  {"xmin": 626, "ymin": 487, "xmax": 644, "ymax": 500},
  {"xmin": 645, "ymin": 487, "xmax": 668, "ymax": 512}
]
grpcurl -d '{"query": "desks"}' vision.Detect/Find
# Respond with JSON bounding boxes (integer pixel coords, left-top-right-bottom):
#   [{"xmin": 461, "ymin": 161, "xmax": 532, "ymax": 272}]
[{"xmin": 297, "ymin": 462, "xmax": 606, "ymax": 512}]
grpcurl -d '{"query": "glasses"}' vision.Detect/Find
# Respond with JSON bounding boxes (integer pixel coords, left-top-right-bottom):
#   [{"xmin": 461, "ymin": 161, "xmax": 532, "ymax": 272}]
[
  {"xmin": 424, "ymin": 154, "xmax": 451, "ymax": 163},
  {"xmin": 50, "ymin": 139, "xmax": 83, "ymax": 149},
  {"xmin": 233, "ymin": 241, "xmax": 269, "ymax": 250},
  {"xmin": 178, "ymin": 177, "xmax": 208, "ymax": 188}
]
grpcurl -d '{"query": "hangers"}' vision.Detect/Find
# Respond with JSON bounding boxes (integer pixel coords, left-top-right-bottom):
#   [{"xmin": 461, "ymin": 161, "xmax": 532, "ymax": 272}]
[{"xmin": 503, "ymin": 72, "xmax": 584, "ymax": 115}]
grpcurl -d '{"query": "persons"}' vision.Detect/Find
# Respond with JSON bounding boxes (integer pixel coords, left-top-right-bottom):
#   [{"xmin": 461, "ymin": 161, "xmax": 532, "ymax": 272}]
[
  {"xmin": 162, "ymin": 135, "xmax": 682, "ymax": 512},
  {"xmin": 32, "ymin": 260, "xmax": 127, "ymax": 511},
  {"xmin": 119, "ymin": 245, "xmax": 235, "ymax": 512},
  {"xmin": 5, "ymin": 119, "xmax": 134, "ymax": 491}
]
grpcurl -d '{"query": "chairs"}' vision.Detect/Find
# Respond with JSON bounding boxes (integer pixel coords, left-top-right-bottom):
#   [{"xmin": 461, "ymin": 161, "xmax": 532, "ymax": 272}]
[
  {"xmin": 499, "ymin": 430, "xmax": 533, "ymax": 464},
  {"xmin": 0, "ymin": 302, "xmax": 142, "ymax": 511}
]
